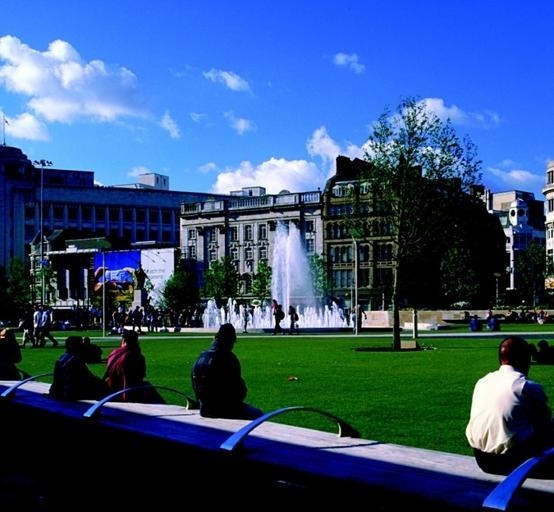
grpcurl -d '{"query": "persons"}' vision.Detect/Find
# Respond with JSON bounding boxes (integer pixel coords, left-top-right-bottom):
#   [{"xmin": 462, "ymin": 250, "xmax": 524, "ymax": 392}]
[
  {"xmin": 272, "ymin": 300, "xmax": 279, "ymax": 313},
  {"xmin": 352, "ymin": 305, "xmax": 356, "ymax": 331},
  {"xmin": 469, "ymin": 315, "xmax": 484, "ymax": 332},
  {"xmin": 48, "ymin": 334, "xmax": 103, "ymax": 399},
  {"xmin": 486, "ymin": 311, "xmax": 501, "ymax": 332},
  {"xmin": 464, "ymin": 311, "xmax": 470, "ymax": 322},
  {"xmin": 94, "ymin": 267, "xmax": 136, "ymax": 293},
  {"xmin": 288, "ymin": 305, "xmax": 300, "ymax": 336},
  {"xmin": 353, "ymin": 304, "xmax": 368, "ymax": 333},
  {"xmin": 503, "ymin": 307, "xmax": 549, "ymax": 324},
  {"xmin": 191, "ymin": 323, "xmax": 266, "ymax": 421},
  {"xmin": 107, "ymin": 330, "xmax": 169, "ymax": 404},
  {"xmin": 1, "ymin": 327, "xmax": 23, "ymax": 380},
  {"xmin": 484, "ymin": 308, "xmax": 494, "ymax": 321},
  {"xmin": 465, "ymin": 335, "xmax": 553, "ymax": 479},
  {"xmin": 132, "ymin": 264, "xmax": 151, "ymax": 290},
  {"xmin": 271, "ymin": 304, "xmax": 287, "ymax": 337},
  {"xmin": 1, "ymin": 302, "xmax": 206, "ymax": 350}
]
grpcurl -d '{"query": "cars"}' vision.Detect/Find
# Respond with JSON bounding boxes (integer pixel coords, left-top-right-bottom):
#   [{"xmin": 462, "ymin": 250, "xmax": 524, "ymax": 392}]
[{"xmin": 451, "ymin": 301, "xmax": 470, "ymax": 310}]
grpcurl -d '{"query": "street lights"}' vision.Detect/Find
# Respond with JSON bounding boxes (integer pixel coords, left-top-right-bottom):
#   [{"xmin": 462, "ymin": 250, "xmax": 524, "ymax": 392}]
[
  {"xmin": 99, "ymin": 246, "xmax": 108, "ymax": 336},
  {"xmin": 32, "ymin": 159, "xmax": 55, "ymax": 306},
  {"xmin": 351, "ymin": 237, "xmax": 360, "ymax": 336}
]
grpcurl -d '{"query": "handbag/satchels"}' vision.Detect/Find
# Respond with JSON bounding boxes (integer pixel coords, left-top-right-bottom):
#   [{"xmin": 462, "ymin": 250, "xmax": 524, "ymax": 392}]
[
  {"xmin": 96, "ymin": 383, "xmax": 110, "ymax": 399},
  {"xmin": 295, "ymin": 313, "xmax": 298, "ymax": 321}
]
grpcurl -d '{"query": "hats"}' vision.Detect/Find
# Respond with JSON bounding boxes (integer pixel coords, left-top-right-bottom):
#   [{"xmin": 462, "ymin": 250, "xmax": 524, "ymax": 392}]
[{"xmin": 215, "ymin": 324, "xmax": 236, "ymax": 343}]
